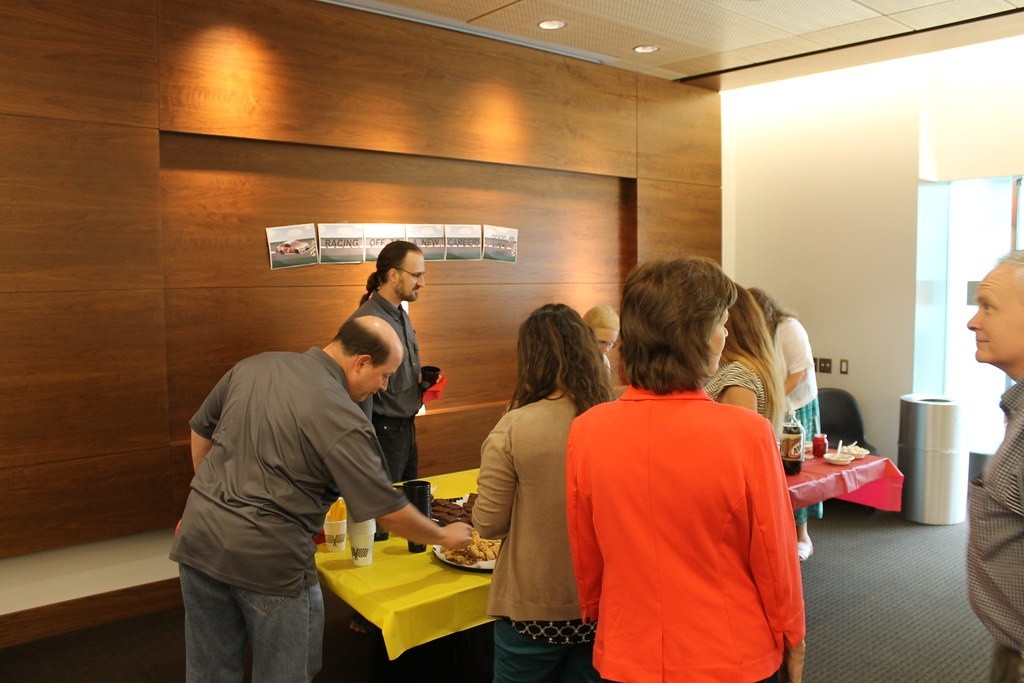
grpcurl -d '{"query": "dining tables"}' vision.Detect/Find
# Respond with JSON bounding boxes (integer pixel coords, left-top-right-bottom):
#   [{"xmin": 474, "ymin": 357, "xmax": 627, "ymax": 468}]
[
  {"xmin": 786, "ymin": 449, "xmax": 904, "ymax": 512},
  {"xmin": 313, "ymin": 469, "xmax": 502, "ymax": 662}
]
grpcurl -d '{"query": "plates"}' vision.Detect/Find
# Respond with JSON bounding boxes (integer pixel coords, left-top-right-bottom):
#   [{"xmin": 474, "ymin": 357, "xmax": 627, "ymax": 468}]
[
  {"xmin": 821, "ymin": 453, "xmax": 855, "ymax": 464},
  {"xmin": 431, "ymin": 545, "xmax": 500, "ymax": 572},
  {"xmin": 839, "ymin": 446, "xmax": 870, "ymax": 459}
]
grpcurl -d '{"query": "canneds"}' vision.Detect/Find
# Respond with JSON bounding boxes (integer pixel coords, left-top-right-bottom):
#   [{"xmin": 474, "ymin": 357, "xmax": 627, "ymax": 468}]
[{"xmin": 812, "ymin": 433, "xmax": 829, "ymax": 458}]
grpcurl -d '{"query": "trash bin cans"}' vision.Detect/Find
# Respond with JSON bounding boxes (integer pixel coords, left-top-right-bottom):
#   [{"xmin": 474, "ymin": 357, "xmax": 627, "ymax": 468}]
[{"xmin": 898, "ymin": 392, "xmax": 969, "ymax": 526}]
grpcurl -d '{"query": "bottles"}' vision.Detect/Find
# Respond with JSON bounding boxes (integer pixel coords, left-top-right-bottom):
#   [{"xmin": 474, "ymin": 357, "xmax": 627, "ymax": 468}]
[{"xmin": 780, "ymin": 410, "xmax": 828, "ymax": 474}]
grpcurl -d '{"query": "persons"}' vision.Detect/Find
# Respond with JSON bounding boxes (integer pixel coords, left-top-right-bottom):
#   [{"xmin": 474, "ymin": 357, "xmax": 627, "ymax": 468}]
[
  {"xmin": 704, "ymin": 282, "xmax": 786, "ymax": 442},
  {"xmin": 967, "ymin": 250, "xmax": 1024, "ymax": 683},
  {"xmin": 746, "ymin": 288, "xmax": 823, "ymax": 561},
  {"xmin": 471, "ymin": 304, "xmax": 617, "ymax": 683},
  {"xmin": 565, "ymin": 251, "xmax": 805, "ymax": 683},
  {"xmin": 355, "ymin": 240, "xmax": 420, "ymax": 484},
  {"xmin": 168, "ymin": 315, "xmax": 478, "ymax": 683},
  {"xmin": 583, "ymin": 305, "xmax": 620, "ymax": 380}
]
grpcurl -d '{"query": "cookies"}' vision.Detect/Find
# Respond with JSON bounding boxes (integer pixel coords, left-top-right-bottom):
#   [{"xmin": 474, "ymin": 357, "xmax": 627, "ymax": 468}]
[{"xmin": 431, "ymin": 493, "xmax": 501, "ymax": 565}]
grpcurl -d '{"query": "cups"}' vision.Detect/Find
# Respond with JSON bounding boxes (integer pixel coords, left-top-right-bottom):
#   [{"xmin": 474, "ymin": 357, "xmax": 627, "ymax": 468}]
[
  {"xmin": 324, "ymin": 520, "xmax": 347, "ymax": 551},
  {"xmin": 404, "ymin": 479, "xmax": 430, "ymax": 552},
  {"xmin": 420, "ymin": 365, "xmax": 441, "ymax": 393},
  {"xmin": 346, "ymin": 511, "xmax": 377, "ymax": 566}
]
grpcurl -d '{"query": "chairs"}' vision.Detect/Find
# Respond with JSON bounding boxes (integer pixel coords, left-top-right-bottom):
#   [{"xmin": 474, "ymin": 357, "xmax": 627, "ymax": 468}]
[{"xmin": 818, "ymin": 388, "xmax": 879, "ymax": 514}]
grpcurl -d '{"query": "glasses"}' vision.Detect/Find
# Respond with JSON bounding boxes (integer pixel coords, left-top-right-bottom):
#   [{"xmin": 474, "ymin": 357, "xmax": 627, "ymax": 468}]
[{"xmin": 396, "ymin": 266, "xmax": 425, "ymax": 278}]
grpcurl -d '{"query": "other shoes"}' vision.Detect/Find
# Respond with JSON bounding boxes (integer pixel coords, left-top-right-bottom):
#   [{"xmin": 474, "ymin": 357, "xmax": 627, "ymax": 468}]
[{"xmin": 797, "ymin": 538, "xmax": 813, "ymax": 561}]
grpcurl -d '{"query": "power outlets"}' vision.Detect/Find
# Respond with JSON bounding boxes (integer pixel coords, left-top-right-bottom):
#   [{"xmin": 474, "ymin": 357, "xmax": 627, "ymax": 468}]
[{"xmin": 814, "ymin": 358, "xmax": 832, "ymax": 373}]
